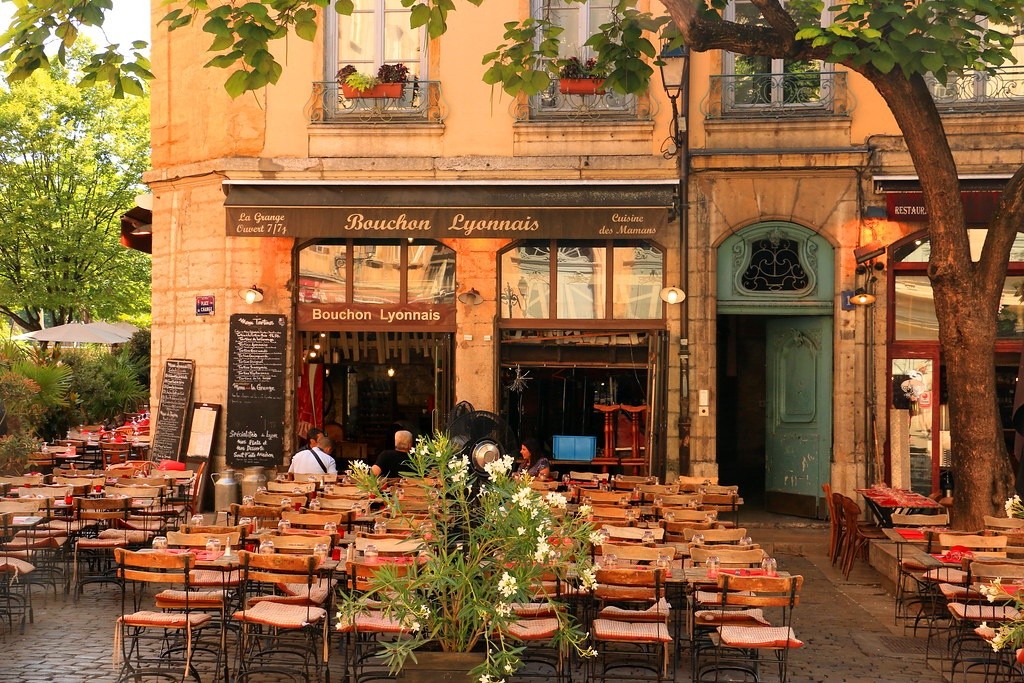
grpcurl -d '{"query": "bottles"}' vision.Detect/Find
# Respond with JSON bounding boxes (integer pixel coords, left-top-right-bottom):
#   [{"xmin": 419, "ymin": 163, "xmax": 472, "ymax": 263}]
[
  {"xmin": 996, "ymin": 374, "xmax": 1015, "ymax": 406},
  {"xmin": 422, "ymin": 399, "xmax": 428, "ymax": 415},
  {"xmin": 210, "ymin": 466, "xmax": 267, "ymax": 515}
]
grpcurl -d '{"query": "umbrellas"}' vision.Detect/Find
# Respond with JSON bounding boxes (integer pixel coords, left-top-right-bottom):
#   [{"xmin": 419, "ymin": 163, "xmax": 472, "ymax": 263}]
[{"xmin": 11, "ymin": 321, "xmax": 141, "ymax": 352}]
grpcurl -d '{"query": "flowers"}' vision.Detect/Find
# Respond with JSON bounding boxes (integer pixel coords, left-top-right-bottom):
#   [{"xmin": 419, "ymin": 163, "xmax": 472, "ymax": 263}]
[
  {"xmin": 332, "ymin": 426, "xmax": 601, "ymax": 683},
  {"xmin": 1003, "ymin": 495, "xmax": 1024, "ymax": 520},
  {"xmin": 979, "ymin": 575, "xmax": 1024, "ymax": 653}
]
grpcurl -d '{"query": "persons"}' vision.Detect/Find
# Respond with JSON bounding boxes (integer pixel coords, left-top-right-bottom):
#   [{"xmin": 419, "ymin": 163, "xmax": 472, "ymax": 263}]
[
  {"xmin": 288, "ymin": 428, "xmax": 337, "ymax": 474},
  {"xmin": 518, "ymin": 438, "xmax": 549, "ymax": 481},
  {"xmin": 370, "ymin": 431, "xmax": 418, "ymax": 478}
]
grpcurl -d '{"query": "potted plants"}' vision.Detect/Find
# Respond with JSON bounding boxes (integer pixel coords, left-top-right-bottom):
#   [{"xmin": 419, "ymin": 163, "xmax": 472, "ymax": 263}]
[
  {"xmin": 558, "ymin": 56, "xmax": 611, "ymax": 94},
  {"xmin": 335, "ymin": 62, "xmax": 410, "ymax": 99}
]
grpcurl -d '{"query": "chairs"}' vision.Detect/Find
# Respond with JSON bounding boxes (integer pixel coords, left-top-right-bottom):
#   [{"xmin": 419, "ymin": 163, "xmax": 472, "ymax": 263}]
[{"xmin": 0, "ymin": 402, "xmax": 1024, "ymax": 683}]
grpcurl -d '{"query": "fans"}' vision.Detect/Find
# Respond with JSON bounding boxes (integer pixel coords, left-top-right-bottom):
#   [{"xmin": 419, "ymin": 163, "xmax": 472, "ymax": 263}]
[{"xmin": 444, "ymin": 410, "xmax": 519, "ymax": 529}]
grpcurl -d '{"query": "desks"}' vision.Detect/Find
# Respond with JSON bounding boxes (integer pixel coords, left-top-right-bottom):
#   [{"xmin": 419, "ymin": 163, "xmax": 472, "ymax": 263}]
[
  {"xmin": 879, "ymin": 527, "xmax": 981, "ymax": 623},
  {"xmin": 628, "ymin": 567, "xmax": 790, "ymax": 680},
  {"xmin": 132, "ymin": 546, "xmax": 243, "ymax": 666},
  {"xmin": 854, "ymin": 486, "xmax": 945, "ymax": 526},
  {"xmin": 11, "ymin": 516, "xmax": 43, "ymax": 636}
]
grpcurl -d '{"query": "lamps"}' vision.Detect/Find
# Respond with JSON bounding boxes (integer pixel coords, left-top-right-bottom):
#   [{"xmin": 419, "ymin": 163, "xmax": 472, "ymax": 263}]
[
  {"xmin": 238, "ymin": 285, "xmax": 263, "ymax": 304},
  {"xmin": 849, "ymin": 287, "xmax": 877, "ymax": 305},
  {"xmin": 659, "ymin": 285, "xmax": 686, "ymax": 305},
  {"xmin": 458, "ymin": 287, "xmax": 483, "ymax": 310},
  {"xmin": 867, "ymin": 205, "xmax": 888, "ymax": 224},
  {"xmin": 853, "ymin": 239, "xmax": 887, "ymax": 265},
  {"xmin": 131, "ymin": 223, "xmax": 152, "ymax": 236}
]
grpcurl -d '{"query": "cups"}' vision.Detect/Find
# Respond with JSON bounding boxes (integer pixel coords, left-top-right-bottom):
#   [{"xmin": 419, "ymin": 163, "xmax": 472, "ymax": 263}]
[
  {"xmin": 870, "ymin": 482, "xmax": 909, "ymax": 506},
  {"xmin": 563, "ymin": 474, "xmax": 777, "ymax": 577},
  {"xmin": 6, "ymin": 483, "xmax": 102, "ymax": 504},
  {"xmin": 150, "ymin": 474, "xmax": 440, "ymax": 564}
]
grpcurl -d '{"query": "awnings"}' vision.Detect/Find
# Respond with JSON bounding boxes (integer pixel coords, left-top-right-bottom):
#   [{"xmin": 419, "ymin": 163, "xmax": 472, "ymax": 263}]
[
  {"xmin": 875, "ymin": 181, "xmax": 1024, "ymax": 222},
  {"xmin": 224, "ymin": 183, "xmax": 675, "ymax": 237}
]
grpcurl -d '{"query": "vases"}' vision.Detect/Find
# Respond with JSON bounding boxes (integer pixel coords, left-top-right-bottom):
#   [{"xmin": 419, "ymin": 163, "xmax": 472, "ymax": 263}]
[{"xmin": 401, "ymin": 641, "xmax": 493, "ymax": 683}]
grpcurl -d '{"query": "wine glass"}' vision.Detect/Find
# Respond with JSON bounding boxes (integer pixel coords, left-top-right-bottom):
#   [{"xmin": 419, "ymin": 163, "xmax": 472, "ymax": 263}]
[{"xmin": 40, "ymin": 412, "xmax": 149, "ymax": 455}]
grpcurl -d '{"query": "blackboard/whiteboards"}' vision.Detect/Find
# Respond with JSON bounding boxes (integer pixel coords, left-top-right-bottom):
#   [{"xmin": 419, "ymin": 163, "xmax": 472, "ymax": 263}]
[
  {"xmin": 226, "ymin": 314, "xmax": 287, "ymax": 469},
  {"xmin": 149, "ymin": 358, "xmax": 195, "ymax": 468}
]
